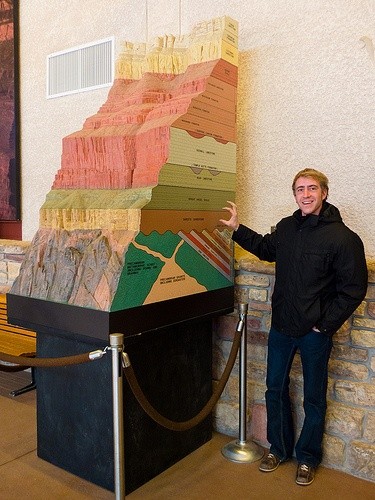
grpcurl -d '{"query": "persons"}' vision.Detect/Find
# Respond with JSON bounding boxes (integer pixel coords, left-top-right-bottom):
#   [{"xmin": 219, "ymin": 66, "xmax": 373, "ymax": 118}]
[{"xmin": 219, "ymin": 168, "xmax": 368, "ymax": 486}]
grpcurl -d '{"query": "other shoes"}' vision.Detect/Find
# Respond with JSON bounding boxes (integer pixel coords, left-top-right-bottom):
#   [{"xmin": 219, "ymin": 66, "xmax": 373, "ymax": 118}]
[
  {"xmin": 295, "ymin": 464, "xmax": 315, "ymax": 485},
  {"xmin": 258, "ymin": 452, "xmax": 292, "ymax": 473}
]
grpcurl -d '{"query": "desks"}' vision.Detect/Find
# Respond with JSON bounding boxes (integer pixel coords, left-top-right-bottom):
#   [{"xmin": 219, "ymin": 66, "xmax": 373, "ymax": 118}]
[{"xmin": 5, "ymin": 286, "xmax": 237, "ymax": 496}]
